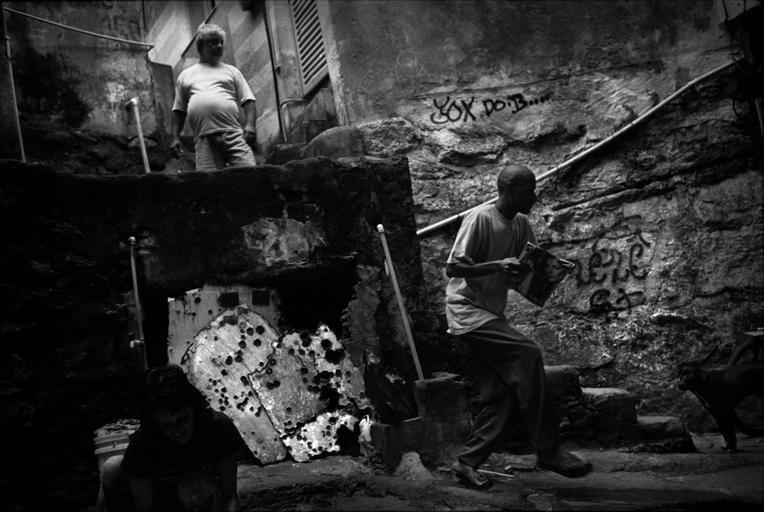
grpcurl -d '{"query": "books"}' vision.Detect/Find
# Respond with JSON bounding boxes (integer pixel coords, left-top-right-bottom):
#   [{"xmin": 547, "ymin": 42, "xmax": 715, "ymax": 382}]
[{"xmin": 502, "ymin": 242, "xmax": 575, "ymax": 308}]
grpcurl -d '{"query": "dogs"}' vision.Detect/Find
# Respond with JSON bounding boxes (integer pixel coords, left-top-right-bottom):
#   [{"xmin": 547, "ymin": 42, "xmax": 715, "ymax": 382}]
[{"xmin": 676, "ymin": 363, "xmax": 764, "ymax": 452}]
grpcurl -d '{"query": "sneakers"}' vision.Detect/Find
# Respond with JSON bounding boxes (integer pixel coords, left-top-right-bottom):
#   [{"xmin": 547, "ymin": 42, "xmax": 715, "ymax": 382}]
[
  {"xmin": 536, "ymin": 451, "xmax": 593, "ymax": 478},
  {"xmin": 452, "ymin": 462, "xmax": 489, "ymax": 489}
]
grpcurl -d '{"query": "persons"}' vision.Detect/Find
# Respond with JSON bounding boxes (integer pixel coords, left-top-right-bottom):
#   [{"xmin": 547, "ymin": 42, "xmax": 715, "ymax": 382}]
[
  {"xmin": 169, "ymin": 24, "xmax": 256, "ymax": 171},
  {"xmin": 446, "ymin": 166, "xmax": 591, "ymax": 490},
  {"xmin": 544, "ymin": 258, "xmax": 567, "ymax": 282},
  {"xmin": 99, "ymin": 365, "xmax": 245, "ymax": 512}
]
